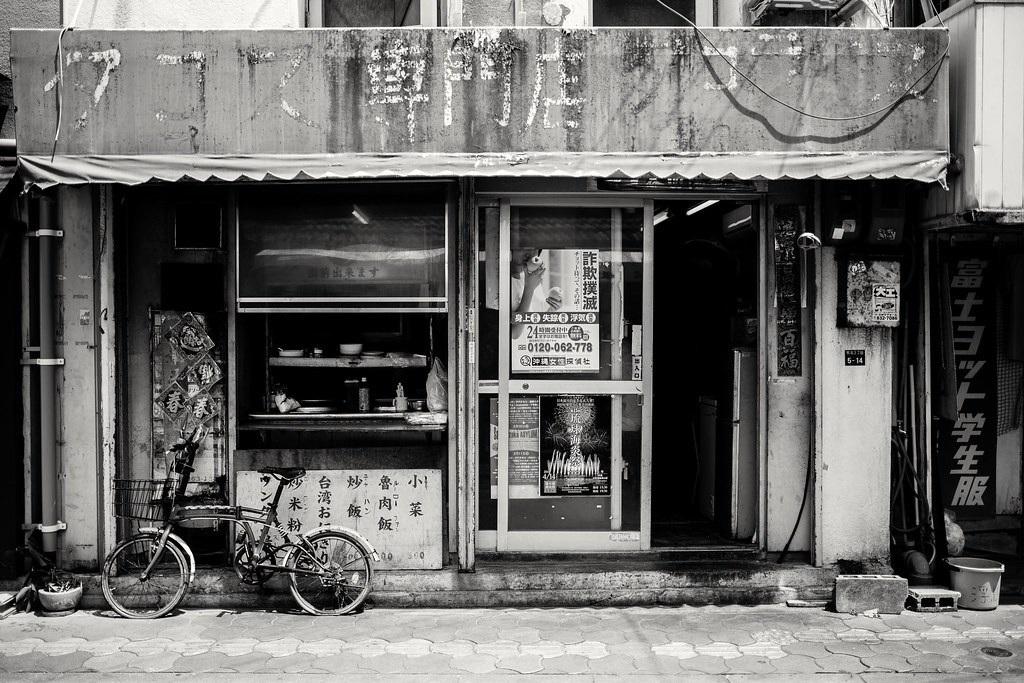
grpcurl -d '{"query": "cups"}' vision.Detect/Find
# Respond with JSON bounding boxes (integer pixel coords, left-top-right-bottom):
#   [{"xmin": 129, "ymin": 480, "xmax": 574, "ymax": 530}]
[{"xmin": 393, "ymin": 397, "xmax": 408, "ymax": 413}]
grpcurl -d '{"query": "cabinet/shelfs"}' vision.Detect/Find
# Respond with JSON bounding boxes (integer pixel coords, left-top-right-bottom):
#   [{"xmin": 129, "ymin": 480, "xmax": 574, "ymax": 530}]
[{"xmin": 263, "ymin": 313, "xmax": 435, "ymax": 419}]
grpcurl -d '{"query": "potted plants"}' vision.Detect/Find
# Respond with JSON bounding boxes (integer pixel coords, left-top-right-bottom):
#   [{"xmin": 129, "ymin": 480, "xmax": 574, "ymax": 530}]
[{"xmin": 38, "ymin": 567, "xmax": 83, "ymax": 617}]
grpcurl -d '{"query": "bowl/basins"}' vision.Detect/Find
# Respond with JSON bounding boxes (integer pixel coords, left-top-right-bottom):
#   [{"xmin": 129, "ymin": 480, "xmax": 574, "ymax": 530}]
[
  {"xmin": 374, "ymin": 398, "xmax": 393, "ymax": 406},
  {"xmin": 279, "ymin": 350, "xmax": 304, "ymax": 357},
  {"xmin": 408, "ymin": 399, "xmax": 426, "ymax": 412}
]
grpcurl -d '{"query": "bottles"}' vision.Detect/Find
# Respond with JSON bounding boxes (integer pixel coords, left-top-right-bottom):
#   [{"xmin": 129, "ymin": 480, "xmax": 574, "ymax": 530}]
[
  {"xmin": 358, "ymin": 377, "xmax": 370, "ymax": 413},
  {"xmin": 314, "ymin": 350, "xmax": 322, "ymax": 357}
]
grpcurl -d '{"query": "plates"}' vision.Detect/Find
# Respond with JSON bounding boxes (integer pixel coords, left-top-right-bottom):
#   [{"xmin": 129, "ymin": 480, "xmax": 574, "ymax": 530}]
[
  {"xmin": 301, "ymin": 400, "xmax": 332, "ymax": 405},
  {"xmin": 360, "ymin": 352, "xmax": 385, "ymax": 359},
  {"xmin": 337, "ymin": 344, "xmax": 362, "ymax": 358}
]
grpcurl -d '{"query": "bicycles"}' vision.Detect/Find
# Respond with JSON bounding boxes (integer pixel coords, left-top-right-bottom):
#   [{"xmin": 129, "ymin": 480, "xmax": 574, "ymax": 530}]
[{"xmin": 101, "ymin": 411, "xmax": 381, "ymax": 620}]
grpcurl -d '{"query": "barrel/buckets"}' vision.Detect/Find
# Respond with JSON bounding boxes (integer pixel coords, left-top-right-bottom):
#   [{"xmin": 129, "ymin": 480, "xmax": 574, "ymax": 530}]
[{"xmin": 942, "ymin": 557, "xmax": 1005, "ymax": 610}]
